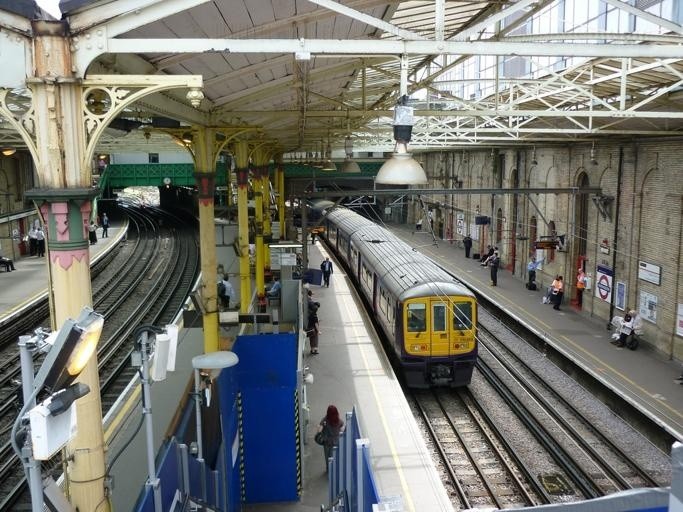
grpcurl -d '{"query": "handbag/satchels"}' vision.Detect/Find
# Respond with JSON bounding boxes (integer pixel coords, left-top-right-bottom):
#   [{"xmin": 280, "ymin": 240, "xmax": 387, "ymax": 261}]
[
  {"xmin": 314, "ymin": 420, "xmax": 328, "ymax": 445},
  {"xmin": 305, "ymin": 327, "xmax": 316, "ymax": 337}
]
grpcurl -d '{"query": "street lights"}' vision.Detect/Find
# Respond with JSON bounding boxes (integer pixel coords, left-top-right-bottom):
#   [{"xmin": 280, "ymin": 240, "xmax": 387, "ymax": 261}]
[
  {"xmin": 15, "ymin": 304, "xmax": 106, "ymax": 512},
  {"xmin": 187, "ymin": 349, "xmax": 242, "ymax": 463}
]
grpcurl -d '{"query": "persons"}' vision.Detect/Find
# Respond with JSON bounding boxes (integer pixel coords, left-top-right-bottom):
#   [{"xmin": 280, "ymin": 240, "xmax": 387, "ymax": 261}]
[
  {"xmin": 87, "ymin": 220, "xmax": 99, "ymax": 246},
  {"xmin": 313, "ymin": 404, "xmax": 345, "ymax": 475},
  {"xmin": 28, "ymin": 226, "xmax": 37, "ymax": 257},
  {"xmin": 0, "ymin": 256, "xmax": 15, "ymax": 272},
  {"xmin": 550, "ymin": 278, "xmax": 559, "ymax": 288},
  {"xmin": 462, "ymin": 233, "xmax": 472, "ymax": 258},
  {"xmin": 262, "ymin": 275, "xmax": 280, "ymax": 298},
  {"xmin": 218, "ymin": 274, "xmax": 235, "ymax": 309},
  {"xmin": 544, "ymin": 275, "xmax": 563, "ymax": 304},
  {"xmin": 614, "ymin": 310, "xmax": 642, "ymax": 347},
  {"xmin": 527, "ymin": 256, "xmax": 546, "ymax": 290},
  {"xmin": 35, "ymin": 228, "xmax": 45, "ymax": 257},
  {"xmin": 576, "ymin": 268, "xmax": 584, "ymax": 307},
  {"xmin": 305, "ymin": 289, "xmax": 322, "ymax": 354},
  {"xmin": 320, "ymin": 256, "xmax": 333, "ymax": 288},
  {"xmin": 100, "ymin": 213, "xmax": 109, "ymax": 237},
  {"xmin": 476, "ymin": 245, "xmax": 500, "ymax": 287}
]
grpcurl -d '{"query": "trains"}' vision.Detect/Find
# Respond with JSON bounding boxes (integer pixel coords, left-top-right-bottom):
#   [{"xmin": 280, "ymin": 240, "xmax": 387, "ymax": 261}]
[{"xmin": 302, "ymin": 194, "xmax": 481, "ymax": 393}]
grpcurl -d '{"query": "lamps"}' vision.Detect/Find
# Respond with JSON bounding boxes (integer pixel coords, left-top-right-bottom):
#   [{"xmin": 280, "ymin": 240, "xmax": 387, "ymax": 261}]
[{"xmin": 321, "ymin": 53, "xmax": 431, "ymax": 186}]
